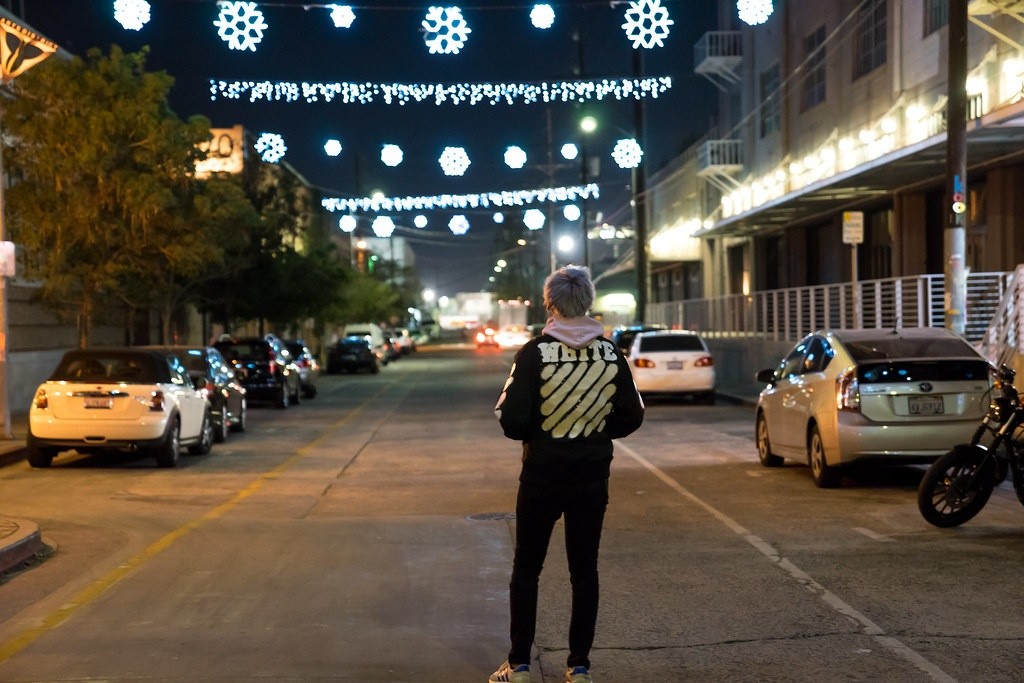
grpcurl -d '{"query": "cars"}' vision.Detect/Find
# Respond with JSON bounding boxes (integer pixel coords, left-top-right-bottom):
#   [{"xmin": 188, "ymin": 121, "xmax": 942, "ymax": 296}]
[
  {"xmin": 474, "ymin": 329, "xmax": 500, "ymax": 347},
  {"xmin": 327, "ymin": 334, "xmax": 381, "ymax": 374},
  {"xmin": 109, "ymin": 343, "xmax": 247, "ymax": 441},
  {"xmin": 345, "ymin": 323, "xmax": 440, "ymax": 365},
  {"xmin": 24, "ymin": 346, "xmax": 216, "ymax": 466},
  {"xmin": 756, "ymin": 327, "xmax": 1009, "ymax": 490},
  {"xmin": 623, "ymin": 329, "xmax": 717, "ymax": 405},
  {"xmin": 279, "ymin": 338, "xmax": 320, "ymax": 399},
  {"xmin": 612, "ymin": 326, "xmax": 666, "ymax": 357}
]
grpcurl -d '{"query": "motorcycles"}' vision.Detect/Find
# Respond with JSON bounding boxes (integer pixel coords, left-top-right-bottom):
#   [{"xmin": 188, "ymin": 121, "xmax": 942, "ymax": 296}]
[{"xmin": 916, "ymin": 343, "xmax": 1024, "ymax": 530}]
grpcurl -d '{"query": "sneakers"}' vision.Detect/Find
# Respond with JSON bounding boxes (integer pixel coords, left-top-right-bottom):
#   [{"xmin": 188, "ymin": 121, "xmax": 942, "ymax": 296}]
[
  {"xmin": 488, "ymin": 660, "xmax": 531, "ymax": 683},
  {"xmin": 566, "ymin": 666, "xmax": 592, "ymax": 683}
]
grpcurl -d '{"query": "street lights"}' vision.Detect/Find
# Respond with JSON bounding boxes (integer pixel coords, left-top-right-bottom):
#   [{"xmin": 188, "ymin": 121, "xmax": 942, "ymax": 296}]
[{"xmin": 578, "ymin": 114, "xmax": 648, "ymax": 323}]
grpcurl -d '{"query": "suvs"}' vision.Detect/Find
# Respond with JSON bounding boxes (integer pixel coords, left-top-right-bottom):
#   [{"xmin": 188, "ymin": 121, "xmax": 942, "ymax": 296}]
[{"xmin": 211, "ymin": 333, "xmax": 302, "ymax": 407}]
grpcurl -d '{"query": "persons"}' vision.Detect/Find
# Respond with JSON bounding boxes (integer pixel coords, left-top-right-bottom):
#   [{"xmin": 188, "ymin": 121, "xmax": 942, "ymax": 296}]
[{"xmin": 486, "ymin": 263, "xmax": 645, "ymax": 683}]
